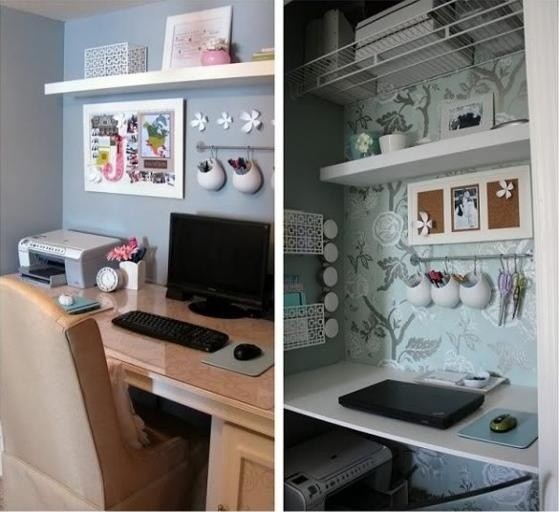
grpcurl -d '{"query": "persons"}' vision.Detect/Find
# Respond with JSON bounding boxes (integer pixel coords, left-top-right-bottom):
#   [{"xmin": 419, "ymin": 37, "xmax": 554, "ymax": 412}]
[{"xmin": 455, "ymin": 189, "xmax": 474, "ymax": 216}]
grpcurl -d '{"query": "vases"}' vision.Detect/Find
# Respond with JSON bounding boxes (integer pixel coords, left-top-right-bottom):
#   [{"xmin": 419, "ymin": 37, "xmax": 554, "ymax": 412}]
[
  {"xmin": 118, "ymin": 260, "xmax": 146, "ymax": 290},
  {"xmin": 200, "ymin": 50, "xmax": 232, "ymax": 67}
]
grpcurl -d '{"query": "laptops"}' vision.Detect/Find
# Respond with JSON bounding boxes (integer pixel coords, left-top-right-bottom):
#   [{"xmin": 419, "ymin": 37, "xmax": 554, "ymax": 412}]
[{"xmin": 338, "ymin": 379, "xmax": 484, "ymax": 430}]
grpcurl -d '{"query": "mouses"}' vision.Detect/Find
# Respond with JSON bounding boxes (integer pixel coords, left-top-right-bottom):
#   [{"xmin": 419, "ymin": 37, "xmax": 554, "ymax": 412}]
[
  {"xmin": 234, "ymin": 343, "xmax": 262, "ymax": 360},
  {"xmin": 489, "ymin": 414, "xmax": 517, "ymax": 433}
]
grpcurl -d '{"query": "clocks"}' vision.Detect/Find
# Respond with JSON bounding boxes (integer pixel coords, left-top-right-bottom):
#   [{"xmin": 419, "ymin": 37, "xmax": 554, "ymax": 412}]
[{"xmin": 94, "ymin": 267, "xmax": 123, "ymax": 293}]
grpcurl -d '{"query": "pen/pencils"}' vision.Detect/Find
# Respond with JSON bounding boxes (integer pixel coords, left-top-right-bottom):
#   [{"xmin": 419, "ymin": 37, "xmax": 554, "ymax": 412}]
[
  {"xmin": 228, "ymin": 157, "xmax": 251, "ymax": 175},
  {"xmin": 402, "ymin": 270, "xmax": 472, "ymax": 287},
  {"xmin": 199, "ymin": 159, "xmax": 214, "ymax": 173}
]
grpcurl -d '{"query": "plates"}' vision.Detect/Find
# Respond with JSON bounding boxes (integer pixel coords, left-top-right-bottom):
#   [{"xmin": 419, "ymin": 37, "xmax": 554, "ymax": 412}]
[{"xmin": 462, "ymin": 371, "xmax": 490, "ymax": 388}]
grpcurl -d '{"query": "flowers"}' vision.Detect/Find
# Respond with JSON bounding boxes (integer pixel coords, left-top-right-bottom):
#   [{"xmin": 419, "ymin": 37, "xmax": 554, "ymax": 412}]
[
  {"xmin": 107, "ymin": 236, "xmax": 147, "ymax": 264},
  {"xmin": 204, "ymin": 37, "xmax": 230, "ymax": 52}
]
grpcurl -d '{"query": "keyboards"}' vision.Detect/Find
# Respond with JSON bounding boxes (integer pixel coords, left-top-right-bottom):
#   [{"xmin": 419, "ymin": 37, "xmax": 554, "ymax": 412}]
[{"xmin": 112, "ymin": 310, "xmax": 229, "ymax": 353}]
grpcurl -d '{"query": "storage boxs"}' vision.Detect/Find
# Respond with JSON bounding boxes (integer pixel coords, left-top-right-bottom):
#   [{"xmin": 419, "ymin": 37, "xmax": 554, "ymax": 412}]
[{"xmin": 301, "ymin": 1, "xmax": 523, "ymax": 106}]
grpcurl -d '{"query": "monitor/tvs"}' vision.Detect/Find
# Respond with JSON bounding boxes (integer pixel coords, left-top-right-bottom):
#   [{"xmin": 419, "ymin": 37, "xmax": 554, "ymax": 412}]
[{"xmin": 166, "ymin": 212, "xmax": 271, "ymax": 318}]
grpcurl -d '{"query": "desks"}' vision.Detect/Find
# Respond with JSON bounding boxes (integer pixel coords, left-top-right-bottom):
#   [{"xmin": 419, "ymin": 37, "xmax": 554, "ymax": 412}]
[
  {"xmin": 283, "ymin": 359, "xmax": 539, "ymax": 477},
  {"xmin": 0, "ymin": 271, "xmax": 274, "ymax": 511}
]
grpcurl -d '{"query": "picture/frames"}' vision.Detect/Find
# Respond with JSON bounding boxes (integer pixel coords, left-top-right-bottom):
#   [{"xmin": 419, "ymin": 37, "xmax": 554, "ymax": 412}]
[
  {"xmin": 160, "ymin": 4, "xmax": 233, "ymax": 70},
  {"xmin": 82, "ymin": 97, "xmax": 186, "ymax": 200},
  {"xmin": 406, "ymin": 164, "xmax": 534, "ymax": 247},
  {"xmin": 438, "ymin": 92, "xmax": 494, "ymax": 138}
]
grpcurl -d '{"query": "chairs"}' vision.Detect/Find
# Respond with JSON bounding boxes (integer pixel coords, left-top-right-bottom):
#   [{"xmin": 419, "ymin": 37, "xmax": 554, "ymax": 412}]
[{"xmin": 0, "ymin": 276, "xmax": 194, "ymax": 511}]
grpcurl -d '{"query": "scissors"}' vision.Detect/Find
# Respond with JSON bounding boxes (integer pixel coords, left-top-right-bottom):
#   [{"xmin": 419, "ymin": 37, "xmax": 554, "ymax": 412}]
[{"xmin": 497, "ymin": 272, "xmax": 524, "ymax": 327}]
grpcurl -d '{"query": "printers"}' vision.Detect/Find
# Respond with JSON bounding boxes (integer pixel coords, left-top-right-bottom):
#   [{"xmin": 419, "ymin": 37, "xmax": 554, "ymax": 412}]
[
  {"xmin": 17, "ymin": 229, "xmax": 122, "ymax": 289},
  {"xmin": 284, "ymin": 429, "xmax": 393, "ymax": 511}
]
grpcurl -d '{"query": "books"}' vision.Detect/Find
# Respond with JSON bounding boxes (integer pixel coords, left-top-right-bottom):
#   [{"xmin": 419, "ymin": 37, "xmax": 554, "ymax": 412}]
[{"xmin": 252, "ymin": 48, "xmax": 274, "ymax": 61}]
